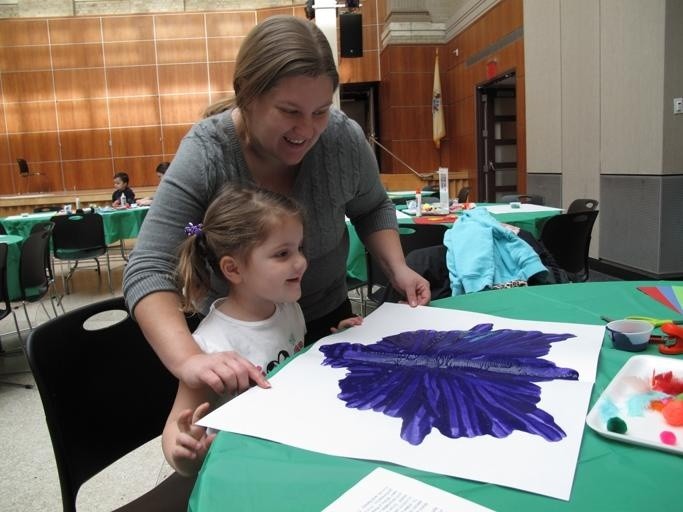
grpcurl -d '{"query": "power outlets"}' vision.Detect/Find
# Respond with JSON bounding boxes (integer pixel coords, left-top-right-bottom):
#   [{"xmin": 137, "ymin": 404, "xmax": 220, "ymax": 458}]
[{"xmin": 673, "ymin": 97, "xmax": 683, "ymax": 114}]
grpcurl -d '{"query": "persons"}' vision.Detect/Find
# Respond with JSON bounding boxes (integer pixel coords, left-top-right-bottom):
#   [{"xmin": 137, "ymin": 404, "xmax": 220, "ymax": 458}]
[
  {"xmin": 122, "ymin": 16, "xmax": 431, "ymax": 395},
  {"xmin": 136, "ymin": 162, "xmax": 170, "ymax": 205},
  {"xmin": 163, "ymin": 183, "xmax": 363, "ymax": 474},
  {"xmin": 112, "ymin": 172, "xmax": 135, "ymax": 206}
]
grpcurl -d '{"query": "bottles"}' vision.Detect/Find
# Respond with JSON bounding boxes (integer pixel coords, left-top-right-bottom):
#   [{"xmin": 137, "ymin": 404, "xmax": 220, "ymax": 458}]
[
  {"xmin": 121, "ymin": 193, "xmax": 126, "ymax": 207},
  {"xmin": 416, "ymin": 187, "xmax": 422, "ymax": 218},
  {"xmin": 76, "ymin": 195, "xmax": 80, "ymax": 209}
]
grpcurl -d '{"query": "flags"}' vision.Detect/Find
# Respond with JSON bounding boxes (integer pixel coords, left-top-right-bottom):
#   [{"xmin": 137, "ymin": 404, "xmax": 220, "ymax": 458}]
[{"xmin": 431, "ymin": 46, "xmax": 447, "ymax": 149}]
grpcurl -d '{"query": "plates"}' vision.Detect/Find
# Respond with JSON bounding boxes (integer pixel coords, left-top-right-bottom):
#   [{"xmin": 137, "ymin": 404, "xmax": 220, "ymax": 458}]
[{"xmin": 585, "ymin": 352, "xmax": 682, "ymax": 456}]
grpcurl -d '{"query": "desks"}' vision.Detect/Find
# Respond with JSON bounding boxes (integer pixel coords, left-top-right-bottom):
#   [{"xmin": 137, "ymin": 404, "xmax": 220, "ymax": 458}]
[
  {"xmin": 344, "ymin": 203, "xmax": 566, "ymax": 291},
  {"xmin": 188, "ymin": 280, "xmax": 682, "ymax": 512},
  {"xmin": 0, "ymin": 236, "xmax": 27, "ymax": 355},
  {"xmin": 0, "ymin": 207, "xmax": 151, "ymax": 277}
]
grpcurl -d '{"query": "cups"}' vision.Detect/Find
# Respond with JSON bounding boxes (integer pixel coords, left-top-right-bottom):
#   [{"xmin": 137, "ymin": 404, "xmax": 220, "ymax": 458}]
[{"xmin": 604, "ymin": 317, "xmax": 657, "ymax": 353}]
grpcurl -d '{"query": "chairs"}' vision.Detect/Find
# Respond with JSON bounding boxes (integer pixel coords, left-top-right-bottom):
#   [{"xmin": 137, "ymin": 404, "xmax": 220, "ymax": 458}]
[
  {"xmin": 25, "ymin": 296, "xmax": 205, "ymax": 511},
  {"xmin": 388, "ymin": 230, "xmax": 554, "ymax": 304},
  {"xmin": 0, "ymin": 243, "xmax": 35, "ymax": 388},
  {"xmin": 11, "ymin": 220, "xmax": 68, "ymax": 330},
  {"xmin": 567, "ymin": 199, "xmax": 598, "ymax": 216},
  {"xmin": 364, "ymin": 223, "xmax": 450, "ymax": 306},
  {"xmin": 50, "ymin": 212, "xmax": 116, "ymax": 291},
  {"xmin": 541, "ymin": 211, "xmax": 599, "ymax": 281},
  {"xmin": 456, "ymin": 187, "xmax": 471, "ymax": 205},
  {"xmin": 16, "ymin": 158, "xmax": 48, "ymax": 195}
]
